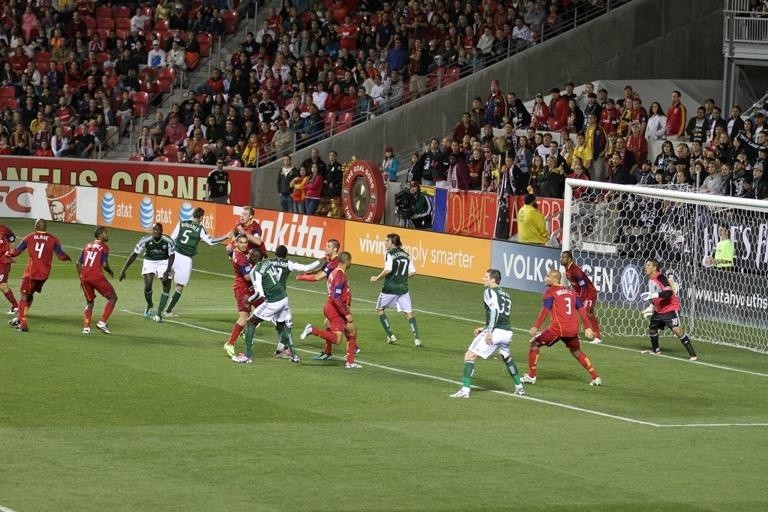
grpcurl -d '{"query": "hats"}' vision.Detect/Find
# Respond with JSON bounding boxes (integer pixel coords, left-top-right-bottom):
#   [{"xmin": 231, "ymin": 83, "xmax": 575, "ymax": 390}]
[
  {"xmin": 672, "ymin": 157, "xmax": 687, "ymax": 165},
  {"xmin": 752, "ymin": 102, "xmax": 761, "ymax": 109},
  {"xmin": 536, "ymin": 92, "xmax": 543, "ymax": 98},
  {"xmin": 641, "ymin": 159, "xmax": 651, "ymax": 166},
  {"xmin": 410, "ymin": 181, "xmax": 419, "ymax": 187},
  {"xmin": 753, "ymin": 164, "xmax": 763, "ymax": 171}
]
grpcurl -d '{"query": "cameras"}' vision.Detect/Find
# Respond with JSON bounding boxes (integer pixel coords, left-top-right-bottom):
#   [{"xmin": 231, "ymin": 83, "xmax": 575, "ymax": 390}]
[{"xmin": 393, "ymin": 188, "xmax": 416, "ymax": 209}]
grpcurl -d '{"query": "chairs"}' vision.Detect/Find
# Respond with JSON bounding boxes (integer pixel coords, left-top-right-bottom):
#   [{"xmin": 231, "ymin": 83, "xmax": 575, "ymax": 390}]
[
  {"xmin": 115, "ymin": 17, "xmax": 131, "ymax": 30},
  {"xmin": 142, "ymin": 7, "xmax": 152, "ymax": 15},
  {"xmin": 323, "ymin": 112, "xmax": 336, "ymax": 135},
  {"xmin": 83, "ymin": 18, "xmax": 96, "ymax": 28},
  {"xmin": 224, "ymin": 10, "xmax": 238, "ymax": 33},
  {"xmin": 0, "ymin": 97, "xmax": 17, "ymax": 111},
  {"xmin": 96, "ymin": 53, "xmax": 111, "ymax": 62},
  {"xmin": 442, "ymin": 67, "xmax": 460, "ymax": 86},
  {"xmin": 36, "ymin": 53, "xmax": 50, "ymax": 62},
  {"xmin": 48, "ymin": 65, "xmax": 64, "ymax": 72},
  {"xmin": 335, "ymin": 112, "xmax": 353, "ymax": 134},
  {"xmin": 97, "ymin": 17, "xmax": 114, "ymax": 28},
  {"xmin": 35, "ymin": 62, "xmax": 47, "ymax": 74},
  {"xmin": 140, "ymin": 68, "xmax": 157, "ymax": 93},
  {"xmin": 226, "ymin": 159, "xmax": 243, "ymax": 167},
  {"xmin": 158, "ymin": 68, "xmax": 175, "ymax": 92},
  {"xmin": 197, "ymin": 32, "xmax": 213, "ymax": 56},
  {"xmin": 430, "ymin": 67, "xmax": 445, "ymax": 81},
  {"xmin": 114, "ymin": 6, "xmax": 131, "ymax": 17},
  {"xmin": 0, "ymin": 86, "xmax": 16, "ymax": 98},
  {"xmin": 180, "ymin": 32, "xmax": 195, "ymax": 42},
  {"xmin": 154, "ymin": 19, "xmax": 169, "ymax": 30},
  {"xmin": 96, "ymin": 6, "xmax": 112, "ymax": 18},
  {"xmin": 133, "ymin": 92, "xmax": 149, "ymax": 117}
]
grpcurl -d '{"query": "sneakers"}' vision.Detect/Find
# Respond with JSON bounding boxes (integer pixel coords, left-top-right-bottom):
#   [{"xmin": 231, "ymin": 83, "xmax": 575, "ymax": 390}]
[
  {"xmin": 231, "ymin": 354, "xmax": 252, "ymax": 365},
  {"xmin": 345, "ymin": 361, "xmax": 363, "ymax": 370},
  {"xmin": 649, "ymin": 347, "xmax": 662, "ymax": 355},
  {"xmin": 312, "ymin": 351, "xmax": 333, "ymax": 360},
  {"xmin": 590, "ymin": 337, "xmax": 602, "ymax": 344},
  {"xmin": 272, "ymin": 350, "xmax": 287, "ymax": 359},
  {"xmin": 513, "ymin": 383, "xmax": 526, "ymax": 396},
  {"xmin": 414, "ymin": 339, "xmax": 423, "ymax": 347},
  {"xmin": 288, "ymin": 354, "xmax": 301, "ymax": 362},
  {"xmin": 83, "ymin": 327, "xmax": 91, "ymax": 334},
  {"xmin": 300, "ymin": 323, "xmax": 313, "ymax": 341},
  {"xmin": 344, "ymin": 345, "xmax": 360, "ymax": 358},
  {"xmin": 590, "ymin": 376, "xmax": 602, "ymax": 387},
  {"xmin": 689, "ymin": 353, "xmax": 700, "ymax": 361},
  {"xmin": 223, "ymin": 341, "xmax": 236, "ymax": 358},
  {"xmin": 96, "ymin": 320, "xmax": 112, "ymax": 335},
  {"xmin": 153, "ymin": 314, "xmax": 162, "ymax": 322},
  {"xmin": 163, "ymin": 311, "xmax": 179, "ymax": 317},
  {"xmin": 385, "ymin": 334, "xmax": 397, "ymax": 344},
  {"xmin": 450, "ymin": 387, "xmax": 471, "ymax": 399},
  {"xmin": 144, "ymin": 303, "xmax": 154, "ymax": 319},
  {"xmin": 519, "ymin": 372, "xmax": 538, "ymax": 385}
]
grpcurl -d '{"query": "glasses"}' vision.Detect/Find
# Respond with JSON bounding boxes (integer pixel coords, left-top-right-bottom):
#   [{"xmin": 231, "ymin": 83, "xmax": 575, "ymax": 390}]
[{"xmin": 218, "ymin": 161, "xmax": 224, "ymax": 164}]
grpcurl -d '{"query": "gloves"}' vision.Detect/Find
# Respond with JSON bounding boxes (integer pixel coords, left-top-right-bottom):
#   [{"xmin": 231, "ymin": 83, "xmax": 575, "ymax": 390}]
[
  {"xmin": 640, "ymin": 291, "xmax": 660, "ymax": 302},
  {"xmin": 641, "ymin": 303, "xmax": 656, "ymax": 318}
]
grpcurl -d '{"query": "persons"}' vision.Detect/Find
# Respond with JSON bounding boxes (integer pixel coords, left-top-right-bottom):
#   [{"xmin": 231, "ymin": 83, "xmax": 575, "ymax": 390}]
[
  {"xmin": 742, "ymin": 119, "xmax": 754, "ymax": 140},
  {"xmin": 748, "ymin": 162, "xmax": 767, "ymax": 200},
  {"xmin": 186, "ymin": 117, "xmax": 207, "ymax": 138},
  {"xmin": 282, "ymin": 71, "xmax": 298, "ymax": 96},
  {"xmin": 755, "ymin": 145, "xmax": 767, "ymax": 162},
  {"xmin": 676, "ymin": 143, "xmax": 689, "ymax": 161},
  {"xmin": 126, "ymin": 40, "xmax": 185, "ymax": 112},
  {"xmin": 242, "ymin": 247, "xmax": 263, "ymax": 310},
  {"xmin": 324, "ymin": 83, "xmax": 344, "ymax": 114},
  {"xmin": 646, "ymin": 102, "xmax": 668, "ymax": 142},
  {"xmin": 271, "ymin": 120, "xmax": 295, "ymax": 156},
  {"xmin": 0, "ymin": 1, "xmax": 126, "ymax": 157},
  {"xmin": 258, "ymin": 121, "xmax": 274, "ymax": 167},
  {"xmin": 185, "ymin": 129, "xmax": 208, "ymax": 153},
  {"xmin": 432, "ymin": 147, "xmax": 449, "ymax": 189},
  {"xmin": 584, "ymin": 92, "xmax": 602, "ymax": 125},
  {"xmin": 296, "ymin": 70, "xmax": 309, "ymax": 86},
  {"xmin": 244, "ymin": 132, "xmax": 268, "ymax": 168},
  {"xmin": 712, "ymin": 157, "xmax": 730, "ymax": 176},
  {"xmin": 452, "ymin": 142, "xmax": 459, "ymax": 152},
  {"xmin": 690, "ymin": 161, "xmax": 707, "ymax": 185},
  {"xmin": 611, "ymin": 151, "xmax": 631, "ymax": 187},
  {"xmin": 496, "ymin": 123, "xmax": 519, "ymax": 159},
  {"xmin": 731, "ymin": 137, "xmax": 747, "ymax": 156},
  {"xmin": 301, "ymin": 94, "xmax": 318, "ymax": 113},
  {"xmin": 343, "ymin": 71, "xmax": 357, "ymax": 88},
  {"xmin": 485, "ymin": 80, "xmax": 508, "ymax": 127},
  {"xmin": 560, "ymin": 251, "xmax": 603, "ymax": 345},
  {"xmin": 452, "ymin": 112, "xmax": 479, "ymax": 142},
  {"xmin": 466, "ymin": 149, "xmax": 483, "ymax": 191},
  {"xmin": 585, "ymin": 112, "xmax": 608, "ymax": 184},
  {"xmin": 231, "ymin": 244, "xmax": 327, "ymax": 364},
  {"xmin": 755, "ymin": 132, "xmax": 767, "ymax": 146},
  {"xmin": 725, "ymin": 105, "xmax": 744, "ymax": 136},
  {"xmin": 598, "ymin": 89, "xmax": 608, "ymax": 108},
  {"xmin": 706, "ymin": 225, "xmax": 734, "ymax": 271},
  {"xmin": 296, "ymin": 239, "xmax": 361, "ymax": 360},
  {"xmin": 197, "ymin": 87, "xmax": 205, "ymax": 102},
  {"xmin": 240, "ymin": 107, "xmax": 256, "ymax": 122},
  {"xmin": 300, "ymin": 251, "xmax": 363, "ymax": 369},
  {"xmin": 572, "ymin": 156, "xmax": 589, "ymax": 180},
  {"xmin": 294, "ymin": 83, "xmax": 310, "ymax": 102},
  {"xmin": 285, "ymin": 95, "xmax": 302, "ymax": 111},
  {"xmin": 214, "ymin": 92, "xmax": 225, "ymax": 104},
  {"xmin": 230, "ymin": 68, "xmax": 247, "ymax": 92},
  {"xmin": 203, "ymin": 144, "xmax": 216, "ymax": 164},
  {"xmin": 164, "ymin": 207, "xmax": 233, "ymax": 316},
  {"xmin": 407, "ymin": 154, "xmax": 419, "ymax": 183},
  {"xmin": 527, "ymin": 155, "xmax": 546, "ymax": 195},
  {"xmin": 567, "ymin": 165, "xmax": 588, "ymax": 201},
  {"xmin": 242, "ymin": 120, "xmax": 256, "ymax": 143},
  {"xmin": 507, "ymin": 91, "xmax": 531, "ymax": 129},
  {"xmin": 446, "ymin": 153, "xmax": 471, "ymax": 191},
  {"xmin": 460, "ymin": 135, "xmax": 470, "ymax": 155},
  {"xmin": 206, "ymin": 68, "xmax": 224, "ymax": 91},
  {"xmin": 235, "ymin": 139, "xmax": 245, "ymax": 154},
  {"xmin": 324, "ymin": 150, "xmax": 343, "ymax": 196},
  {"xmin": 548, "ymin": 86, "xmax": 568, "ymax": 132},
  {"xmin": 470, "ymin": 136, "xmax": 476, "ymax": 146},
  {"xmin": 229, "ymin": 93, "xmax": 244, "ymax": 106},
  {"xmin": 162, "ymin": 136, "xmax": 179, "ymax": 162},
  {"xmin": 705, "ymin": 149, "xmax": 716, "ymax": 162},
  {"xmin": 369, "ymin": 233, "xmax": 421, "ymax": 347},
  {"xmin": 325, "ymin": 72, "xmax": 343, "ymax": 90},
  {"xmin": 351, "ymin": 1, "xmax": 594, "ymax": 73},
  {"xmin": 223, "ymin": 105, "xmax": 241, "ymax": 120},
  {"xmin": 226, "ymin": 155, "xmax": 240, "ymax": 168},
  {"xmin": 751, "ymin": 113, "xmax": 768, "ymax": 132},
  {"xmin": 223, "ymin": 120, "xmax": 239, "ymax": 155},
  {"xmin": 300, "ymin": 148, "xmax": 326, "ymax": 175},
  {"xmin": 281, "ymin": 109, "xmax": 290, "ymax": 120},
  {"xmin": 578, "ymin": 83, "xmax": 594, "ymax": 102},
  {"xmin": 531, "ymin": 92, "xmax": 549, "ymax": 131},
  {"xmin": 560, "ymin": 140, "xmax": 573, "ymax": 163},
  {"xmin": 654, "ymin": 141, "xmax": 677, "ymax": 170},
  {"xmin": 514, "ymin": 136, "xmax": 531, "ymax": 171},
  {"xmin": 468, "ymin": 95, "xmax": 487, "ymax": 129},
  {"xmin": 472, "ymin": 141, "xmax": 482, "ymax": 151},
  {"xmin": 290, "ymin": 107, "xmax": 308, "ymax": 141},
  {"xmin": 216, "ymin": 139, "xmax": 226, "ymax": 162},
  {"xmin": 685, "ymin": 106, "xmax": 708, "ymax": 142},
  {"xmin": 146, "ymin": 111, "xmax": 165, "ymax": 143},
  {"xmin": 541, "ymin": 156, "xmax": 564, "ymax": 198},
  {"xmin": 705, "ymin": 94, "xmax": 715, "ymax": 112},
  {"xmin": 715, "ymin": 134, "xmax": 731, "ymax": 159},
  {"xmin": 382, "ymin": 145, "xmax": 399, "ymax": 182},
  {"xmin": 707, "ymin": 107, "xmax": 726, "ymax": 133},
  {"xmin": 640, "ymin": 259, "xmax": 698, "ymax": 361},
  {"xmin": 230, "ymin": 205, "xmax": 268, "ymax": 258},
  {"xmin": 559, "ymin": 131, "xmax": 570, "ymax": 141},
  {"xmin": 310, "ymin": 82, "xmax": 329, "ymax": 111},
  {"xmin": 339, "ymin": 85, "xmax": 359, "ymax": 117},
  {"xmin": 482, "ymin": 125, "xmax": 494, "ymax": 149},
  {"xmin": 564, "ymin": 82, "xmax": 577, "ymax": 97},
  {"xmin": 488, "ymin": 154, "xmax": 500, "ymax": 193},
  {"xmin": 700, "ymin": 161, "xmax": 724, "ymax": 196},
  {"xmin": 637, "ymin": 161, "xmax": 656, "ymax": 186},
  {"xmin": 729, "ymin": 159, "xmax": 751, "ymax": 199},
  {"xmin": 632, "ymin": 99, "xmax": 649, "ymax": 135},
  {"xmin": 211, "ymin": 104, "xmax": 225, "ymax": 126},
  {"xmin": 480, "ymin": 148, "xmax": 493, "ymax": 192},
  {"xmin": 534, "ymin": 133, "xmax": 558, "ymax": 155},
  {"xmin": 502, "ymin": 158, "xmax": 526, "ymax": 193},
  {"xmin": 668, "ymin": 160, "xmax": 688, "ymax": 185},
  {"xmin": 220, "ymin": 0, "xmax": 351, "ymax": 69},
  {"xmin": 135, "ymin": 126, "xmax": 159, "ymax": 161},
  {"xmin": 159, "ymin": 113, "xmax": 186, "ymax": 148},
  {"xmin": 516, "ymin": 194, "xmax": 547, "ymax": 248},
  {"xmin": 260, "ymin": 69, "xmax": 279, "ymax": 97},
  {"xmin": 713, "ymin": 125, "xmax": 725, "ymax": 138},
  {"xmin": 748, "ymin": 103, "xmax": 764, "ymax": 113},
  {"xmin": 626, "ymin": 121, "xmax": 648, "ymax": 163},
  {"xmin": 187, "ymin": 102, "xmax": 206, "ymax": 122},
  {"xmin": 303, "ymin": 164, "xmax": 323, "ymax": 215},
  {"xmin": 5, "ymin": 217, "xmax": 72, "ymax": 330},
  {"xmin": 566, "ymin": 99, "xmax": 583, "ymax": 132},
  {"xmin": 205, "ymin": 158, "xmax": 228, "ymax": 204},
  {"xmin": 608, "ymin": 137, "xmax": 640, "ymax": 175},
  {"xmin": 359, "ymin": 70, "xmax": 374, "ymax": 95},
  {"xmin": 257, "ymin": 90, "xmax": 280, "ymax": 122},
  {"xmin": 573, "ymin": 132, "xmax": 593, "ymax": 169},
  {"xmin": 519, "ymin": 270, "xmax": 602, "ymax": 387},
  {"xmin": 442, "ymin": 137, "xmax": 451, "ymax": 155},
  {"xmin": 384, "ymin": 70, "xmax": 404, "ymax": 110},
  {"xmin": 545, "ymin": 141, "xmax": 567, "ymax": 166},
  {"xmin": 689, "ymin": 140, "xmax": 703, "ymax": 159},
  {"xmin": 526, "ymin": 128, "xmax": 535, "ymax": 145},
  {"xmin": 654, "ymin": 170, "xmax": 666, "ymax": 185},
  {"xmin": 720, "ymin": 163, "xmax": 733, "ymax": 197},
  {"xmin": 423, "ymin": 141, "xmax": 431, "ymax": 153},
  {"xmin": 288, "ymin": 165, "xmax": 309, "ymax": 214},
  {"xmin": 192, "ymin": 144, "xmax": 203, "ymax": 161},
  {"xmin": 246, "ymin": 93, "xmax": 257, "ymax": 106},
  {"xmin": 357, "ymin": 87, "xmax": 374, "ymax": 125},
  {"xmin": 600, "ymin": 99, "xmax": 619, "ymax": 132},
  {"xmin": 296, "ymin": 104, "xmax": 325, "ymax": 150},
  {"xmin": 407, "ymin": 182, "xmax": 434, "ymax": 233},
  {"xmin": 449, "ymin": 269, "xmax": 527, "ymax": 399},
  {"xmin": 201, "ymin": 93, "xmax": 213, "ymax": 115},
  {"xmin": 675, "ymin": 171, "xmax": 689, "ymax": 191},
  {"xmin": 371, "ymin": 75, "xmax": 388, "ymax": 112},
  {"xmin": 206, "ymin": 115, "xmax": 224, "ymax": 144},
  {"xmin": 619, "ymin": 99, "xmax": 633, "ymax": 136},
  {"xmin": 221, "ymin": 233, "xmax": 265, "ymax": 358},
  {"xmin": 738, "ymin": 147, "xmax": 752, "ymax": 170},
  {"xmin": 75, "ymin": 226, "xmax": 118, "ymax": 335},
  {"xmin": 166, "ymin": 104, "xmax": 184, "ymax": 122},
  {"xmin": 195, "ymin": 154, "xmax": 204, "ymax": 165},
  {"xmin": 126, "ymin": 0, "xmax": 225, "ymax": 40},
  {"xmin": 624, "ymin": 85, "xmax": 633, "ymax": 100},
  {"xmin": 277, "ymin": 154, "xmax": 297, "ymax": 212},
  {"xmin": 178, "ymin": 89, "xmax": 196, "ymax": 113},
  {"xmin": 412, "ymin": 138, "xmax": 442, "ymax": 187},
  {"xmin": 176, "ymin": 149, "xmax": 190, "ymax": 164},
  {"xmin": 119, "ymin": 223, "xmax": 175, "ymax": 323},
  {"xmin": 666, "ymin": 91, "xmax": 685, "ymax": 140},
  {"xmin": 0, "ymin": 225, "xmax": 19, "ymax": 315},
  {"xmin": 247, "ymin": 69, "xmax": 260, "ymax": 93},
  {"xmin": 529, "ymin": 132, "xmax": 543, "ymax": 152},
  {"xmin": 222, "ymin": 71, "xmax": 232, "ymax": 90}
]
